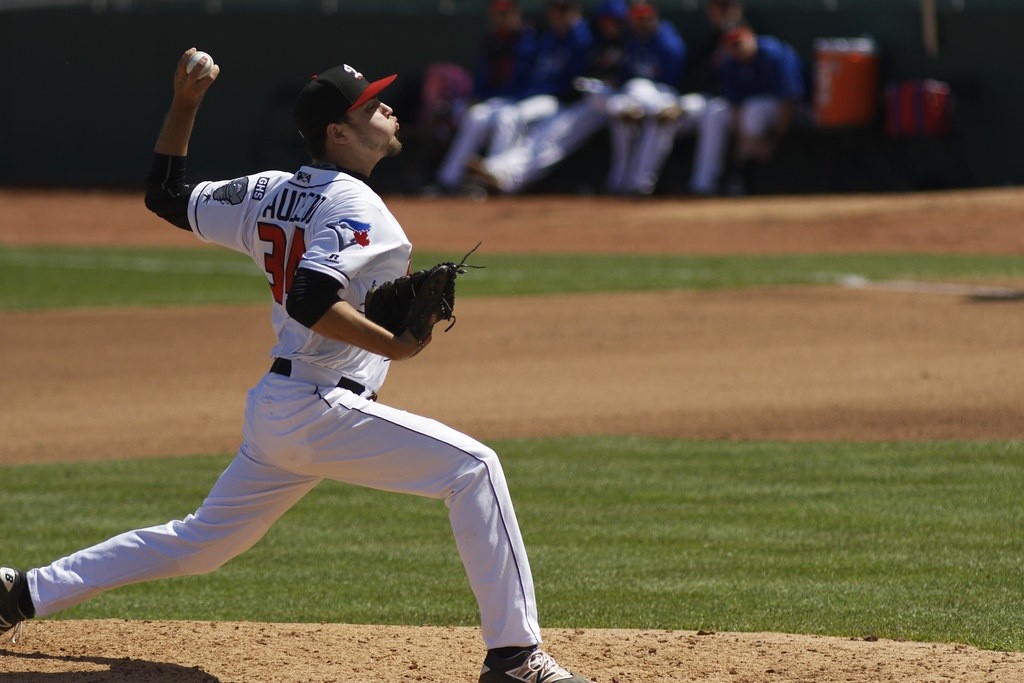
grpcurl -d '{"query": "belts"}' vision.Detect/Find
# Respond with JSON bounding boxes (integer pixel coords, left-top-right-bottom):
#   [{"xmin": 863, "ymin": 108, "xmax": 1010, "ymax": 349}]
[{"xmin": 268, "ymin": 358, "xmax": 378, "ymax": 402}]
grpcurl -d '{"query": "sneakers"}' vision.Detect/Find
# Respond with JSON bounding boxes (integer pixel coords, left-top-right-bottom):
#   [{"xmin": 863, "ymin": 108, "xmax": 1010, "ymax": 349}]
[
  {"xmin": 0, "ymin": 566, "xmax": 30, "ymax": 644},
  {"xmin": 478, "ymin": 649, "xmax": 594, "ymax": 683}
]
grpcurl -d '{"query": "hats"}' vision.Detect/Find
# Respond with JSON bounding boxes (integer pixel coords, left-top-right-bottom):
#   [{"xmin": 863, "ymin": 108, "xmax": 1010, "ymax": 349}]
[{"xmin": 292, "ymin": 64, "xmax": 398, "ymax": 142}]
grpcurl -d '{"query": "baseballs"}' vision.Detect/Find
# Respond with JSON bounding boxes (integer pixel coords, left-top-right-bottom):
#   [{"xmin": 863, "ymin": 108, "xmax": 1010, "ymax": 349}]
[{"xmin": 183, "ymin": 49, "xmax": 216, "ymax": 80}]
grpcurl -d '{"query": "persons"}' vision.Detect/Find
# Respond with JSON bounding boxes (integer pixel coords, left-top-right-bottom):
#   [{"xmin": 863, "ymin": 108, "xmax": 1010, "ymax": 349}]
[
  {"xmin": 0, "ymin": 49, "xmax": 589, "ymax": 683},
  {"xmin": 416, "ymin": 0, "xmax": 800, "ymax": 199}
]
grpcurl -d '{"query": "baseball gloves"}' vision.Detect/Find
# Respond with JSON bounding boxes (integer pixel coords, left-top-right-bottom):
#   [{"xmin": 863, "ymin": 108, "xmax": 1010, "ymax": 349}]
[{"xmin": 361, "ymin": 259, "xmax": 454, "ymax": 337}]
